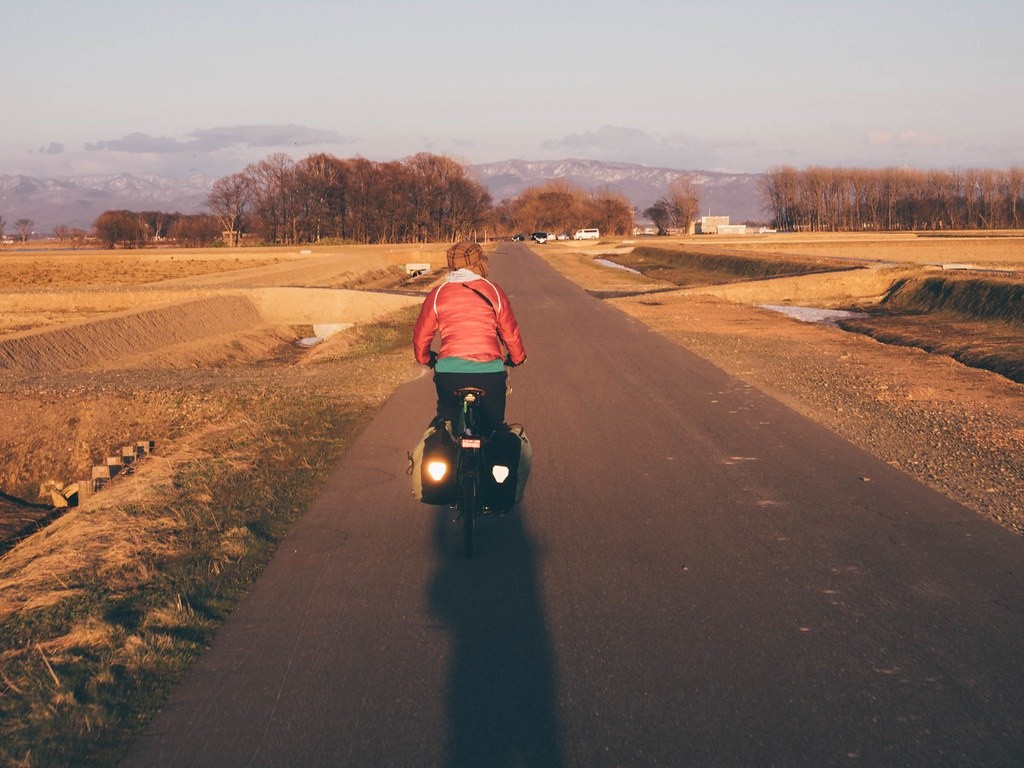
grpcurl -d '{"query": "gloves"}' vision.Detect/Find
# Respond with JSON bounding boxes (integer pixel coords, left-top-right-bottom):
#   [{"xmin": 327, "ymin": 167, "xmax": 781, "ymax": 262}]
[
  {"xmin": 504, "ymin": 353, "xmax": 526, "ymax": 368},
  {"xmin": 425, "ymin": 351, "xmax": 438, "ymax": 368}
]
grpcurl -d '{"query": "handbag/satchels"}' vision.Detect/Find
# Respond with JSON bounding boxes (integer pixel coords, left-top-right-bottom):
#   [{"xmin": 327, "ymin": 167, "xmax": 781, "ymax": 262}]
[{"xmin": 406, "ymin": 418, "xmax": 533, "ymax": 514}]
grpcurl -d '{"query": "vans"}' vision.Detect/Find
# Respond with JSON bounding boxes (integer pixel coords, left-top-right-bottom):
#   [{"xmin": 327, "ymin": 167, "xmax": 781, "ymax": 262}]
[{"xmin": 573, "ymin": 228, "xmax": 600, "ymax": 241}]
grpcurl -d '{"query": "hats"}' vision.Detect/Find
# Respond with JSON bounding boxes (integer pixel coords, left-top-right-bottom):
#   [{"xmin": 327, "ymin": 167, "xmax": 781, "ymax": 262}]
[{"xmin": 447, "ymin": 240, "xmax": 488, "ymax": 274}]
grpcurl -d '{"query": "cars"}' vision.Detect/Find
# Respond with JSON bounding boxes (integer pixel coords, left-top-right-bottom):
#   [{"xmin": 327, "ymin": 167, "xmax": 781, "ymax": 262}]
[
  {"xmin": 512, "ymin": 234, "xmax": 525, "ymax": 242},
  {"xmin": 530, "ymin": 231, "xmax": 570, "ymax": 244}
]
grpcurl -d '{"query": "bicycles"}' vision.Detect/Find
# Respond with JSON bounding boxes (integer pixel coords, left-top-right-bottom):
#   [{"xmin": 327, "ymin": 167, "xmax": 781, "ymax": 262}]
[{"xmin": 430, "ymin": 356, "xmax": 528, "ymax": 560}]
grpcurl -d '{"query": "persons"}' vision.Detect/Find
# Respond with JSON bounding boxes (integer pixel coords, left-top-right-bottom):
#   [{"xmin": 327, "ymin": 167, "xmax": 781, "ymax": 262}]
[{"xmin": 411, "ymin": 241, "xmax": 526, "ymax": 514}]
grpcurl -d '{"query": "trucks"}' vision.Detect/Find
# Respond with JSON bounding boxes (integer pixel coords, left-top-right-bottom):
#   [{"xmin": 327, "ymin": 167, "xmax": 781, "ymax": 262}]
[{"xmin": 758, "ymin": 226, "xmax": 776, "ymax": 234}]
